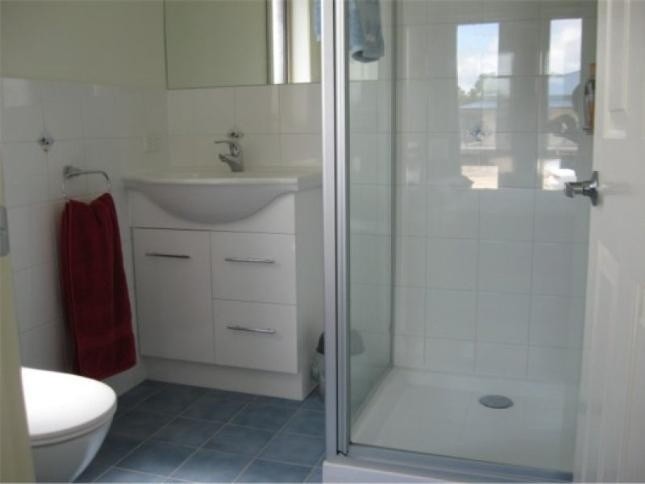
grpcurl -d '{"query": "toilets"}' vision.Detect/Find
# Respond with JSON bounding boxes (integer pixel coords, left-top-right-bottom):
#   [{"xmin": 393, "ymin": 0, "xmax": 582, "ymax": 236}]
[{"xmin": 20, "ymin": 366, "xmax": 117, "ymax": 483}]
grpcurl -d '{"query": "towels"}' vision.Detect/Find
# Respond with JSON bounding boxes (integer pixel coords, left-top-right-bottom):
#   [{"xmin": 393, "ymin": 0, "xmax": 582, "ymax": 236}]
[
  {"xmin": 346, "ymin": 2, "xmax": 388, "ymax": 67},
  {"xmin": 58, "ymin": 193, "xmax": 137, "ymax": 381}
]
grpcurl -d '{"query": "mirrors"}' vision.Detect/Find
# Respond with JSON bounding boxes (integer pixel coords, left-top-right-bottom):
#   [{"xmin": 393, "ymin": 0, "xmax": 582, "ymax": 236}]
[{"xmin": 162, "ymin": 1, "xmax": 326, "ymax": 92}]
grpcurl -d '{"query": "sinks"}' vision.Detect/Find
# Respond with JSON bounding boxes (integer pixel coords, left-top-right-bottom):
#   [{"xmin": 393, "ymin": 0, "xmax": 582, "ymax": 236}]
[{"xmin": 142, "ymin": 170, "xmax": 290, "ymax": 224}]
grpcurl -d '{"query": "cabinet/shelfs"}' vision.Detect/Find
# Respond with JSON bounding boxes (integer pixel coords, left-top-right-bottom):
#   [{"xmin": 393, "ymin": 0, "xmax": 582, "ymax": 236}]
[{"xmin": 131, "ymin": 227, "xmax": 326, "ymax": 401}]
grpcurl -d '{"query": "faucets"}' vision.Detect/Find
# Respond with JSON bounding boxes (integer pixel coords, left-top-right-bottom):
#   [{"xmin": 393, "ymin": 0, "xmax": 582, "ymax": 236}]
[{"xmin": 214, "ymin": 130, "xmax": 244, "ymax": 172}]
